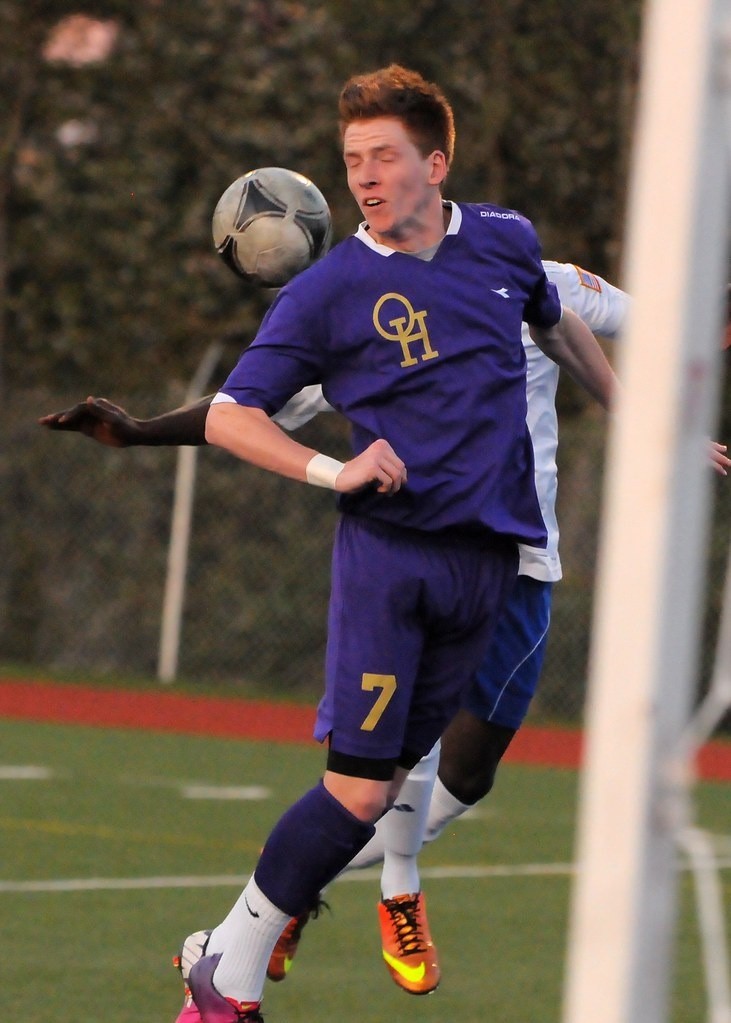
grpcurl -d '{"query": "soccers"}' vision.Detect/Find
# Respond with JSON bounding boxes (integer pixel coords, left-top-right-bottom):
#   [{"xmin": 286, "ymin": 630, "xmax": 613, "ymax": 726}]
[{"xmin": 213, "ymin": 165, "xmax": 333, "ymax": 291}]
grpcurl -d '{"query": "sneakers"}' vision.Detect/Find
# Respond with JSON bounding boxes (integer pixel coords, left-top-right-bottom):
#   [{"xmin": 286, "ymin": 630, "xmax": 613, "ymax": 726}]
[
  {"xmin": 173, "ymin": 929, "xmax": 268, "ymax": 1023},
  {"xmin": 376, "ymin": 888, "xmax": 441, "ymax": 996},
  {"xmin": 266, "ymin": 892, "xmax": 331, "ymax": 983}
]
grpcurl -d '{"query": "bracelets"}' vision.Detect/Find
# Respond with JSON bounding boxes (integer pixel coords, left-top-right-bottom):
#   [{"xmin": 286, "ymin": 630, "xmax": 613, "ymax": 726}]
[{"xmin": 306, "ymin": 453, "xmax": 346, "ymax": 490}]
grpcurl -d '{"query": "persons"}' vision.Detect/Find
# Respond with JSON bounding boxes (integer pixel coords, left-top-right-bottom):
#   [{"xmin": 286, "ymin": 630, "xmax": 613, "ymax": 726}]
[{"xmin": 39, "ymin": 66, "xmax": 731, "ymax": 1023}]
